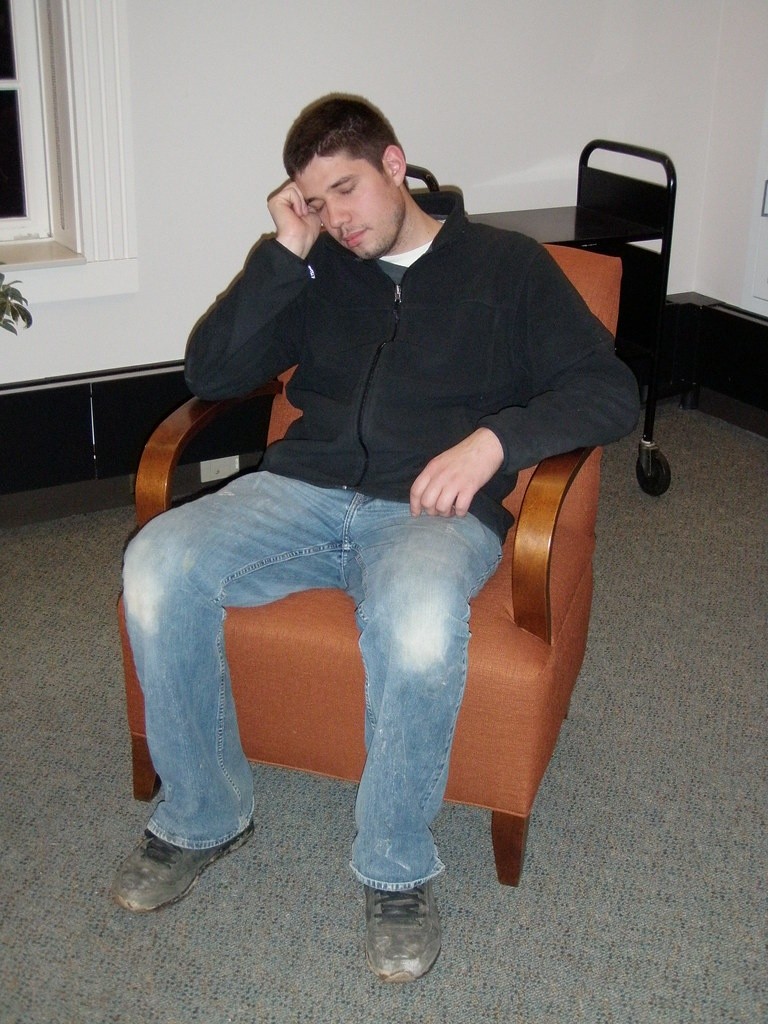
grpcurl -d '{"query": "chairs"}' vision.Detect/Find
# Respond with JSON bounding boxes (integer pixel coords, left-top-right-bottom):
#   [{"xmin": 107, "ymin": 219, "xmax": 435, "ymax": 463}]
[{"xmin": 116, "ymin": 242, "xmax": 622, "ymax": 889}]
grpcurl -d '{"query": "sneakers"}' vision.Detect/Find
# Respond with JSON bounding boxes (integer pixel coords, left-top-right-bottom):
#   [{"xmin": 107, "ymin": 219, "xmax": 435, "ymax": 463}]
[
  {"xmin": 364, "ymin": 882, "xmax": 440, "ymax": 983},
  {"xmin": 111, "ymin": 814, "xmax": 253, "ymax": 913}
]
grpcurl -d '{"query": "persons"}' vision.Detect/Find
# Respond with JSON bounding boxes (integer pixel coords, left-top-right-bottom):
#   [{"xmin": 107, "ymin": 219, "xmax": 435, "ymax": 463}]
[{"xmin": 110, "ymin": 92, "xmax": 642, "ymax": 982}]
